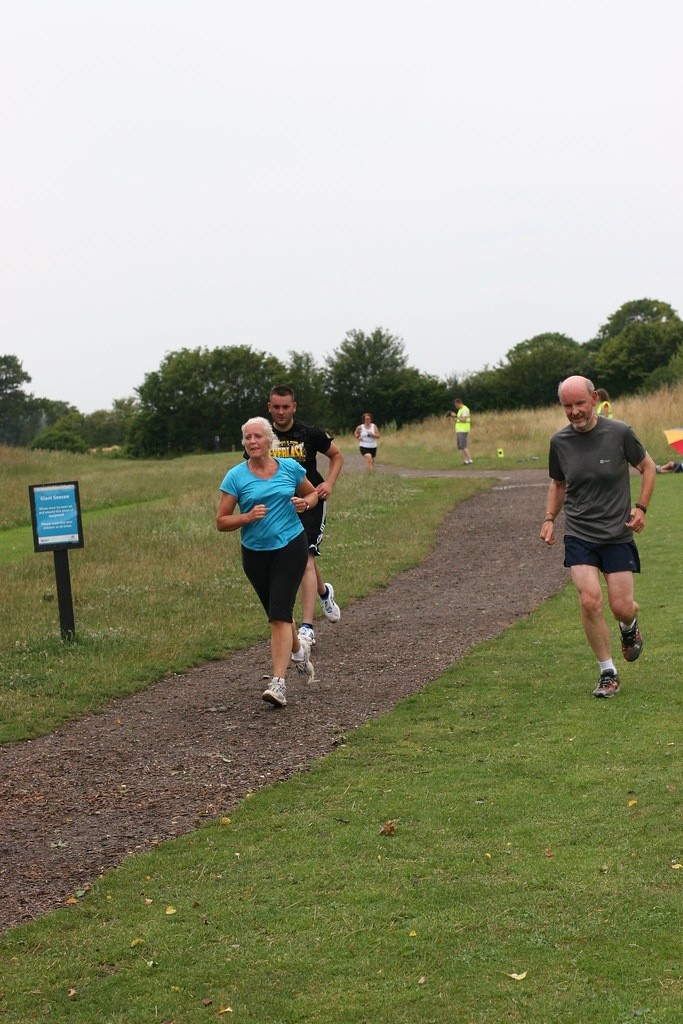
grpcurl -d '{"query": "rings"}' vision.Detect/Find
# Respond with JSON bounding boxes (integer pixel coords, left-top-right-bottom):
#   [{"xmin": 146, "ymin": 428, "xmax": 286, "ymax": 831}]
[
  {"xmin": 324, "ymin": 494, "xmax": 327, "ymax": 497},
  {"xmin": 299, "ymin": 510, "xmax": 300, "ymax": 512}
]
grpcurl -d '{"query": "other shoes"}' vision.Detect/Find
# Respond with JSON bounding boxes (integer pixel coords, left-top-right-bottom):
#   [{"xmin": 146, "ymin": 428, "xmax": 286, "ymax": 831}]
[{"xmin": 656, "ymin": 465, "xmax": 661, "ymax": 473}]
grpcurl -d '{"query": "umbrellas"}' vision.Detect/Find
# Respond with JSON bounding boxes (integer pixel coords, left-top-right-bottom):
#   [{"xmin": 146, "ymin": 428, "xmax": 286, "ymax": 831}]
[{"xmin": 662, "ymin": 428, "xmax": 683, "ymax": 459}]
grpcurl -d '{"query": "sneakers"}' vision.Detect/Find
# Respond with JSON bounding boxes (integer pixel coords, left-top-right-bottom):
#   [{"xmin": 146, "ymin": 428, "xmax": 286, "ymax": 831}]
[
  {"xmin": 297, "ymin": 627, "xmax": 316, "ymax": 647},
  {"xmin": 592, "ymin": 667, "xmax": 622, "ymax": 698},
  {"xmin": 290, "ymin": 640, "xmax": 315, "ymax": 685},
  {"xmin": 318, "ymin": 582, "xmax": 341, "ymax": 623},
  {"xmin": 617, "ymin": 617, "xmax": 644, "ymax": 662},
  {"xmin": 261, "ymin": 680, "xmax": 288, "ymax": 707},
  {"xmin": 464, "ymin": 459, "xmax": 473, "ymax": 464}
]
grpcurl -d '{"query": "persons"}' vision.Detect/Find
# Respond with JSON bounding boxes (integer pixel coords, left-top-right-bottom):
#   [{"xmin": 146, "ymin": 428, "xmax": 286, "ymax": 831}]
[
  {"xmin": 655, "ymin": 460, "xmax": 683, "ymax": 473},
  {"xmin": 540, "ymin": 375, "xmax": 656, "ymax": 698},
  {"xmin": 451, "ymin": 398, "xmax": 473, "ymax": 464},
  {"xmin": 354, "ymin": 413, "xmax": 380, "ymax": 470},
  {"xmin": 216, "ymin": 385, "xmax": 344, "ymax": 706}
]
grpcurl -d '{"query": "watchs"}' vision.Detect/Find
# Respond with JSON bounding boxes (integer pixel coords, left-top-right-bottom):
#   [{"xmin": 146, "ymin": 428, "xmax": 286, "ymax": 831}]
[{"xmin": 304, "ymin": 498, "xmax": 309, "ymax": 511}]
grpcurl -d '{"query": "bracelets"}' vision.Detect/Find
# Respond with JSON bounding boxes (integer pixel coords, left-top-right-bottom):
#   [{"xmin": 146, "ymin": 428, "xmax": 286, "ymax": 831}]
[
  {"xmin": 635, "ymin": 503, "xmax": 647, "ymax": 513},
  {"xmin": 542, "ymin": 512, "xmax": 554, "ymax": 522}
]
